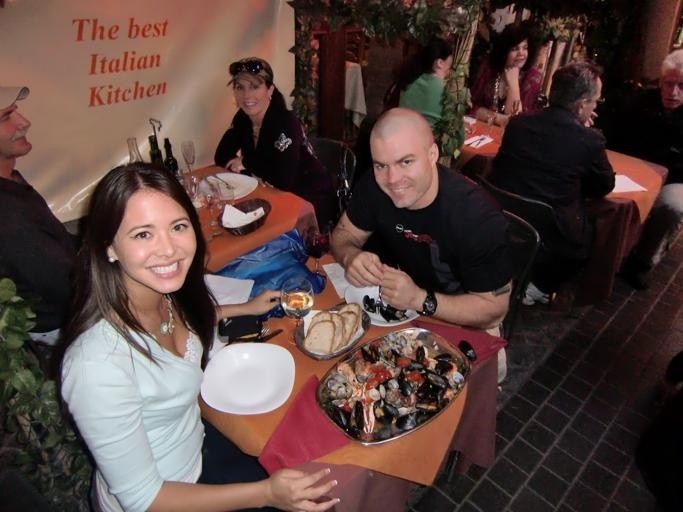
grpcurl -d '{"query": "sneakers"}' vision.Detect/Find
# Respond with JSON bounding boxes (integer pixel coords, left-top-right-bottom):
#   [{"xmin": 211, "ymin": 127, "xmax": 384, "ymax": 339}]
[{"xmin": 520, "ymin": 283, "xmax": 556, "ymax": 306}]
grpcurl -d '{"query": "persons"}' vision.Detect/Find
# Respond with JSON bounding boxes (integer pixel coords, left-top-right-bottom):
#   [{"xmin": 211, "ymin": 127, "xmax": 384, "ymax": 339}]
[
  {"xmin": 52, "ymin": 162, "xmax": 340, "ymax": 512},
  {"xmin": 329, "ymin": 107, "xmax": 518, "ymax": 330},
  {"xmin": 489, "ymin": 62, "xmax": 615, "ymax": 306},
  {"xmin": 391, "ymin": 38, "xmax": 464, "ymax": 169},
  {"xmin": 466, "ymin": 24, "xmax": 544, "ymax": 126},
  {"xmin": 214, "ymin": 55, "xmax": 339, "ymax": 233},
  {"xmin": 0, "ymin": 85, "xmax": 77, "ymax": 376},
  {"xmin": 602, "ymin": 49, "xmax": 683, "ymax": 290}
]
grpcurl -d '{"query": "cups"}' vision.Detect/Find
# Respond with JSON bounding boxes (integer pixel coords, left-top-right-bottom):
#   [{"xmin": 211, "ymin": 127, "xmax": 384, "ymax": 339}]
[{"xmin": 179, "ymin": 167, "xmax": 233, "ymax": 236}]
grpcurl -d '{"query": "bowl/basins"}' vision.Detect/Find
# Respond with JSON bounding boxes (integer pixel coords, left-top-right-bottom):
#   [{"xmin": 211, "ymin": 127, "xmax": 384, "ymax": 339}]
[{"xmin": 216, "ymin": 197, "xmax": 272, "ymax": 236}]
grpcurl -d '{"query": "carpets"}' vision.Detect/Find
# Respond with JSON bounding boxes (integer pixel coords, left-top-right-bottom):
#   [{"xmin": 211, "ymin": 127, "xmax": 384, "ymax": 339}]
[{"xmin": 496, "ymin": 296, "xmax": 593, "ymax": 415}]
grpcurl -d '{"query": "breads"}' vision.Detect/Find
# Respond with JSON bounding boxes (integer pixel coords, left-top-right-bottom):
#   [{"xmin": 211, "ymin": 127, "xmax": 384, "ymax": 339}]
[{"xmin": 302, "ymin": 302, "xmax": 361, "ymax": 355}]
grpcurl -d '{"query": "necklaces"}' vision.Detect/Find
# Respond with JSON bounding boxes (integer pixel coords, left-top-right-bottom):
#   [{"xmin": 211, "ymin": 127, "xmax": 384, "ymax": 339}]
[{"xmin": 139, "ymin": 295, "xmax": 174, "ymax": 337}]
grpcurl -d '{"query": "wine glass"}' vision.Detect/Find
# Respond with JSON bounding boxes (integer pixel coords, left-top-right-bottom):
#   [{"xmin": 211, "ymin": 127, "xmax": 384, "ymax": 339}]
[
  {"xmin": 281, "ymin": 278, "xmax": 314, "ymax": 344},
  {"xmin": 180, "ymin": 141, "xmax": 194, "ymax": 174},
  {"xmin": 301, "ymin": 225, "xmax": 331, "ymax": 291}
]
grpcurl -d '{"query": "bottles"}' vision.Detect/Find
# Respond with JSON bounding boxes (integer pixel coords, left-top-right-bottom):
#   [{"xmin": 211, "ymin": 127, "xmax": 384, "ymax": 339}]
[{"xmin": 125, "ymin": 136, "xmax": 177, "ymax": 174}]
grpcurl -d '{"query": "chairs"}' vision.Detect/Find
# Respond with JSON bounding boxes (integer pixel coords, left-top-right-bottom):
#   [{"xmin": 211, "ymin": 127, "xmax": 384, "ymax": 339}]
[
  {"xmin": 498, "ymin": 205, "xmax": 542, "ymax": 338},
  {"xmin": 471, "ymin": 169, "xmax": 556, "ymax": 301},
  {"xmin": 302, "ymin": 135, "xmax": 359, "ymax": 232}
]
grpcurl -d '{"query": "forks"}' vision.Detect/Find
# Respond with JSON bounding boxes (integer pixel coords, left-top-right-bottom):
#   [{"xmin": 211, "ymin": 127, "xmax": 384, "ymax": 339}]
[
  {"xmin": 464, "ymin": 138, "xmax": 486, "ymax": 149},
  {"xmin": 209, "ymin": 173, "xmax": 235, "ymax": 191}
]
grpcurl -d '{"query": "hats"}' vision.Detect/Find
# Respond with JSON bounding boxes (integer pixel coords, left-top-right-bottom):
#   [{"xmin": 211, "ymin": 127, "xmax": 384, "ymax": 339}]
[
  {"xmin": 227, "ymin": 57, "xmax": 274, "ymax": 86},
  {"xmin": 0, "ymin": 86, "xmax": 30, "ymax": 111}
]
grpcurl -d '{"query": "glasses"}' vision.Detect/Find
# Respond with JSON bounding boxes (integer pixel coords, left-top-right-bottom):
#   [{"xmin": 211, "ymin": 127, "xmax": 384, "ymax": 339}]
[{"xmin": 229, "ymin": 61, "xmax": 268, "ymax": 76}]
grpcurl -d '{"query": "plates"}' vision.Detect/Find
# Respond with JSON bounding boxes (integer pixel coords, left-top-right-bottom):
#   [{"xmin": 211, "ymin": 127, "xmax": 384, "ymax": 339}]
[
  {"xmin": 344, "ymin": 281, "xmax": 420, "ymax": 328},
  {"xmin": 191, "ymin": 172, "xmax": 258, "ymax": 201},
  {"xmin": 200, "ymin": 341, "xmax": 296, "ymax": 415},
  {"xmin": 293, "ymin": 303, "xmax": 370, "ymax": 361}
]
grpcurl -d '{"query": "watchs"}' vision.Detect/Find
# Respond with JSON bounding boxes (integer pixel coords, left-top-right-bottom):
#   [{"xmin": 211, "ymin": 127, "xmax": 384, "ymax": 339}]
[{"xmin": 422, "ymin": 289, "xmax": 437, "ymax": 317}]
